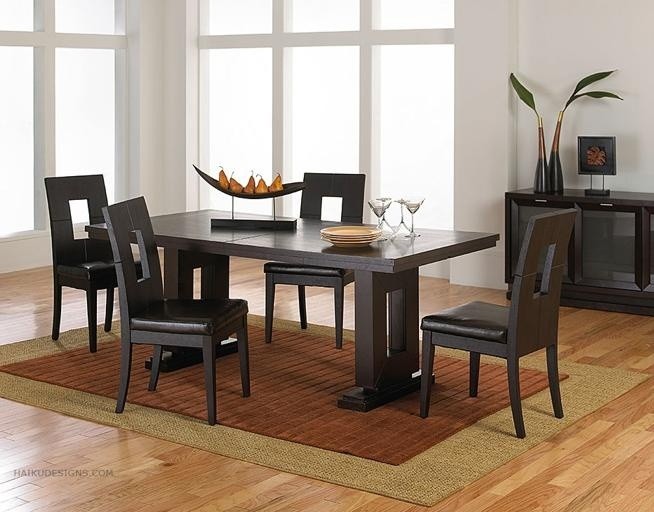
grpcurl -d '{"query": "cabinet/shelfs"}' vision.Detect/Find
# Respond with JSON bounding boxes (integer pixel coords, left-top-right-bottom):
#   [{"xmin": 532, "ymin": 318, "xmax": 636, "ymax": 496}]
[{"xmin": 505, "ymin": 188, "xmax": 654, "ymax": 317}]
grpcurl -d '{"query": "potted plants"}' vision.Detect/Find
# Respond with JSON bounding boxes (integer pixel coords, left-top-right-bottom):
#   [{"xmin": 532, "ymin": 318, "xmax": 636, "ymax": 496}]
[
  {"xmin": 511, "ymin": 72, "xmax": 550, "ymax": 193},
  {"xmin": 549, "ymin": 68, "xmax": 623, "ymax": 191}
]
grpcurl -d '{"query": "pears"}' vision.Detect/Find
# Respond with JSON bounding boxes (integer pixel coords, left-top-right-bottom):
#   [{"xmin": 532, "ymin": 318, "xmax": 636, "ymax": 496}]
[{"xmin": 217, "ymin": 165, "xmax": 283, "ymax": 194}]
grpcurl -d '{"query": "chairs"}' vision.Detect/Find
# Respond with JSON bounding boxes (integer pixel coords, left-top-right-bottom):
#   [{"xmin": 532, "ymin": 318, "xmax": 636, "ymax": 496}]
[
  {"xmin": 102, "ymin": 196, "xmax": 251, "ymax": 426},
  {"xmin": 263, "ymin": 173, "xmax": 366, "ymax": 350},
  {"xmin": 421, "ymin": 209, "xmax": 577, "ymax": 438},
  {"xmin": 44, "ymin": 173, "xmax": 143, "ymax": 353}
]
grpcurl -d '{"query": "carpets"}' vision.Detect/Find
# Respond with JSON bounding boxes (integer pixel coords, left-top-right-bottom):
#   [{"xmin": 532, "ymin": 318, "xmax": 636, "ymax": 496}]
[{"xmin": 1, "ymin": 315, "xmax": 654, "ymax": 508}]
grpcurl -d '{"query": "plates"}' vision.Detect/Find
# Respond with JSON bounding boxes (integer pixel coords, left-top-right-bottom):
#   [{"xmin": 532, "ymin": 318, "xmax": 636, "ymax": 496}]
[{"xmin": 319, "ymin": 226, "xmax": 382, "ymax": 247}]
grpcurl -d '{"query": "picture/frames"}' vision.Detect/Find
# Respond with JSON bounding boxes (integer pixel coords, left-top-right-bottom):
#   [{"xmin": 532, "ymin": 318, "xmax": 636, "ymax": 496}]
[{"xmin": 577, "ymin": 136, "xmax": 616, "ymax": 175}]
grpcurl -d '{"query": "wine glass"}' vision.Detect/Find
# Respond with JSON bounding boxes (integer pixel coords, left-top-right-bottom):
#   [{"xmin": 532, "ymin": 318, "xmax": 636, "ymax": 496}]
[{"xmin": 368, "ymin": 193, "xmax": 427, "ymax": 240}]
